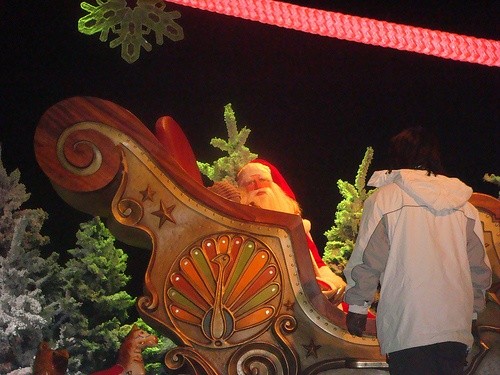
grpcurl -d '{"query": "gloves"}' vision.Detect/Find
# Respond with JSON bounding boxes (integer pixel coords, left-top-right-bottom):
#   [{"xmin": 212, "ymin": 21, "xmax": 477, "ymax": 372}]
[{"xmin": 346, "ymin": 311, "xmax": 368, "ymax": 337}]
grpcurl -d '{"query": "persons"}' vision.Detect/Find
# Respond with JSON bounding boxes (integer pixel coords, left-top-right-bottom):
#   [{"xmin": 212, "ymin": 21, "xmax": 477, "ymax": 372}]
[
  {"xmin": 343, "ymin": 127, "xmax": 493, "ymax": 375},
  {"xmin": 235, "ymin": 159, "xmax": 376, "ymax": 327}
]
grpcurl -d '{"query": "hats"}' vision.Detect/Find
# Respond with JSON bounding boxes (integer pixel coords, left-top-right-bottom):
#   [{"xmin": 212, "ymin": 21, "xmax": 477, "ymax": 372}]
[{"xmin": 237, "ymin": 158, "xmax": 296, "ymax": 201}]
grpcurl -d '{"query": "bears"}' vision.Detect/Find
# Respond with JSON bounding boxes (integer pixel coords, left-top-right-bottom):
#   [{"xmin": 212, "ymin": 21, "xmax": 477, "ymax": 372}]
[{"xmin": 30, "ymin": 341, "xmax": 70, "ymax": 375}]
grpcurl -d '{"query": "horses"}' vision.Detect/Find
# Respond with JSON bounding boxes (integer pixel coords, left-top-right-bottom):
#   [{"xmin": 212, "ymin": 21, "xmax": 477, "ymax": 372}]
[{"xmin": 87, "ymin": 323, "xmax": 159, "ymax": 375}]
